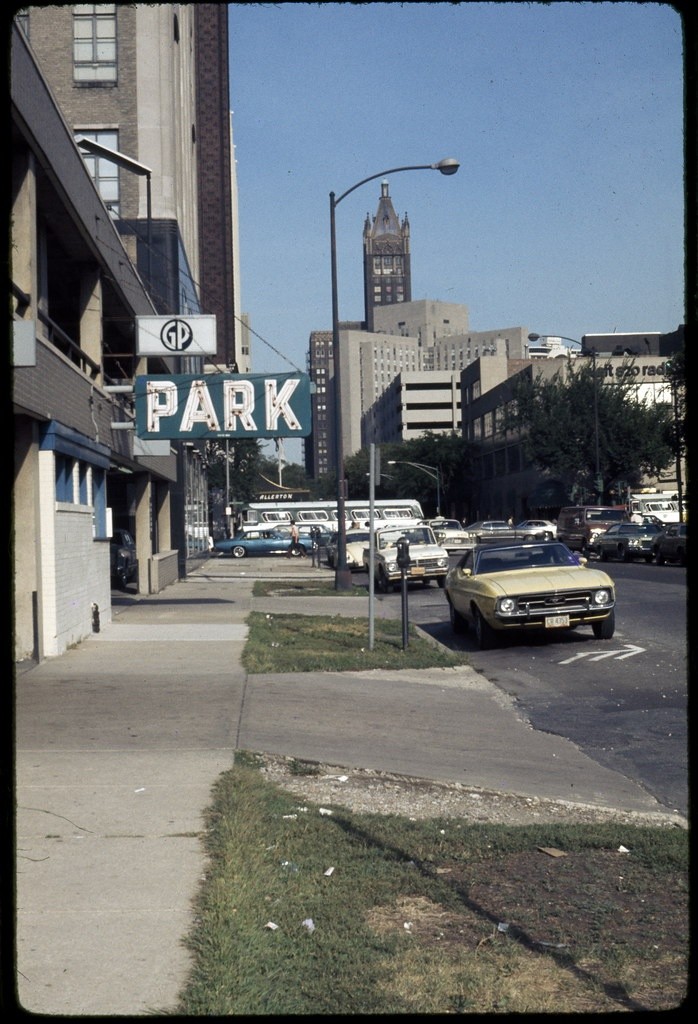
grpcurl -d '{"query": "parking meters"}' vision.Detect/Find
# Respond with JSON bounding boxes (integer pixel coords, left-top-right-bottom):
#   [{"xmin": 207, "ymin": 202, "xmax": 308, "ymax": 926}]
[
  {"xmin": 395, "ymin": 536, "xmax": 411, "ymax": 649},
  {"xmin": 310, "ymin": 527, "xmax": 321, "ymax": 568}
]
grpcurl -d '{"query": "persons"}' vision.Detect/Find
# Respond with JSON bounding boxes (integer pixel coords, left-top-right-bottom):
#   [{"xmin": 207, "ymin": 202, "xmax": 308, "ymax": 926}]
[
  {"xmin": 349, "ymin": 522, "xmax": 359, "ymax": 530},
  {"xmin": 436, "ymin": 513, "xmax": 444, "ymax": 519},
  {"xmin": 508, "ymin": 516, "xmax": 513, "ymax": 526},
  {"xmin": 461, "ymin": 517, "xmax": 469, "ymax": 528},
  {"xmin": 365, "ymin": 522, "xmax": 370, "ymax": 527},
  {"xmin": 285, "ymin": 520, "xmax": 307, "ymax": 559}
]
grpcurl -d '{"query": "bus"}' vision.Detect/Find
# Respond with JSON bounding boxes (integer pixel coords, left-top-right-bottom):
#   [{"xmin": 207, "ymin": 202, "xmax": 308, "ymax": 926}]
[
  {"xmin": 624, "ymin": 494, "xmax": 687, "ymax": 524},
  {"xmin": 226, "ymin": 500, "xmax": 426, "ymax": 538}
]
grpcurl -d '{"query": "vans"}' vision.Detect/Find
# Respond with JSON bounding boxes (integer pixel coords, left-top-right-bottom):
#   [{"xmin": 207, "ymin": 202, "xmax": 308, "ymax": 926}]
[{"xmin": 556, "ymin": 507, "xmax": 632, "ymax": 559}]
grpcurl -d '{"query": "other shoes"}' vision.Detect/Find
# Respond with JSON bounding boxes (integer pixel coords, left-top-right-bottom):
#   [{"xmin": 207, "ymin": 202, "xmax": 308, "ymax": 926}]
[
  {"xmin": 303, "ymin": 555, "xmax": 308, "ymax": 560},
  {"xmin": 284, "ymin": 557, "xmax": 290, "ymax": 560}
]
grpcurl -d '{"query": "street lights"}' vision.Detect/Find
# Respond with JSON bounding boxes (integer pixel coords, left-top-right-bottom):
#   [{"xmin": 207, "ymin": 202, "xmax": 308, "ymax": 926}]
[
  {"xmin": 527, "ymin": 332, "xmax": 602, "ymax": 507},
  {"xmin": 388, "ymin": 460, "xmax": 441, "ymax": 516},
  {"xmin": 333, "ymin": 160, "xmax": 459, "ymax": 591}
]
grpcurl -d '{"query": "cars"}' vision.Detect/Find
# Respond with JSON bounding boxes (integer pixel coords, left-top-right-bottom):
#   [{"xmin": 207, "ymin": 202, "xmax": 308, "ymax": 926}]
[
  {"xmin": 412, "ymin": 516, "xmax": 474, "ymax": 553},
  {"xmin": 212, "ymin": 531, "xmax": 318, "ymax": 558},
  {"xmin": 516, "ymin": 520, "xmax": 556, "ymax": 542},
  {"xmin": 647, "ymin": 526, "xmax": 687, "ymax": 566},
  {"xmin": 326, "ymin": 531, "xmax": 372, "ymax": 570},
  {"xmin": 623, "ymin": 513, "xmax": 667, "ymax": 528},
  {"xmin": 444, "ymin": 541, "xmax": 617, "ymax": 642},
  {"xmin": 272, "ymin": 523, "xmax": 337, "ymax": 547},
  {"xmin": 463, "ymin": 519, "xmax": 538, "ymax": 542},
  {"xmin": 364, "ymin": 525, "xmax": 447, "ymax": 589},
  {"xmin": 109, "ymin": 530, "xmax": 138, "ymax": 583},
  {"xmin": 185, "ymin": 533, "xmax": 208, "ymax": 551},
  {"xmin": 595, "ymin": 521, "xmax": 665, "ymax": 560}
]
